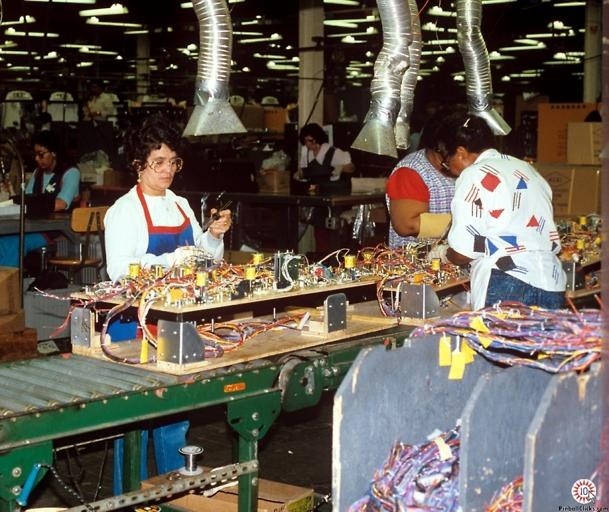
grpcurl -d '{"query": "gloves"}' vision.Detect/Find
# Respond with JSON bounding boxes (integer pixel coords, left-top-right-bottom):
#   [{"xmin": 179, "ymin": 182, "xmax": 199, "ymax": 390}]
[{"xmin": 175, "ymin": 246, "xmax": 209, "ymax": 267}]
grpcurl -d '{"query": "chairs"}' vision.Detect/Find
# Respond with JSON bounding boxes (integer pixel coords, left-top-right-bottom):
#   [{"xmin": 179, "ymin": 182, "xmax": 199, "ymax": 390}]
[{"xmin": 49, "ymin": 206, "xmax": 109, "ymax": 282}]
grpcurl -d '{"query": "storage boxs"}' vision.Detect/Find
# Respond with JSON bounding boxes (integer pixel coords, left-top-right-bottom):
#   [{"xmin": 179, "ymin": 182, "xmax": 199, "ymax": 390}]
[{"xmin": 531, "ymin": 104, "xmax": 601, "ymax": 219}]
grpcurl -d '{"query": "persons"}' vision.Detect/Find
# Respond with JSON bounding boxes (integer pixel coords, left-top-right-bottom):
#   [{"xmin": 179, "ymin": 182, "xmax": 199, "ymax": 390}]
[
  {"xmin": 295, "ymin": 123, "xmax": 354, "ymax": 254},
  {"xmin": 100, "ymin": 114, "xmax": 235, "ymax": 497},
  {"xmin": 82, "ymin": 78, "xmax": 121, "ymax": 171},
  {"xmin": 0, "ymin": 133, "xmax": 81, "ymax": 290},
  {"xmin": 384, "ymin": 103, "xmax": 469, "ymax": 252},
  {"xmin": 426, "ymin": 109, "xmax": 569, "ymax": 315}
]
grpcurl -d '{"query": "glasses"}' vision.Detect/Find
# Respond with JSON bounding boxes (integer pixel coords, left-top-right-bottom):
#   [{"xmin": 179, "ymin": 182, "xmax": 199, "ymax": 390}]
[
  {"xmin": 440, "ymin": 152, "xmax": 450, "ymax": 170},
  {"xmin": 147, "ymin": 157, "xmax": 183, "ymax": 173}
]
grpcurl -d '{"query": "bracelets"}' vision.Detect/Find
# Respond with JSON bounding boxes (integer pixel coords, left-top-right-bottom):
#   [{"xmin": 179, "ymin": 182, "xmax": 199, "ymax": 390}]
[{"xmin": 96, "ymin": 110, "xmax": 101, "ymax": 117}]
